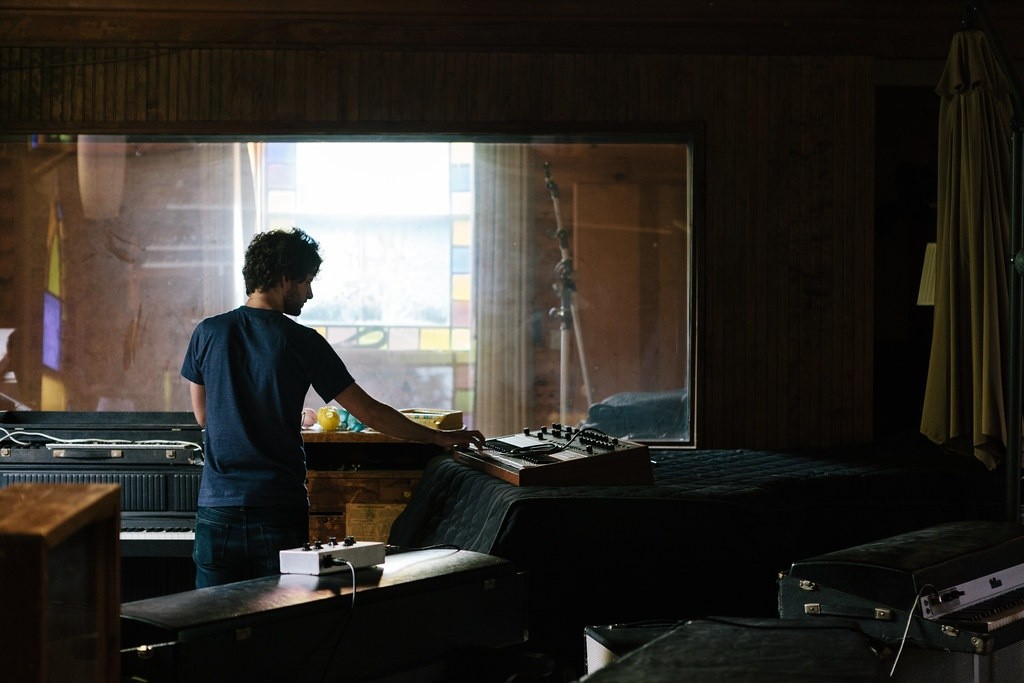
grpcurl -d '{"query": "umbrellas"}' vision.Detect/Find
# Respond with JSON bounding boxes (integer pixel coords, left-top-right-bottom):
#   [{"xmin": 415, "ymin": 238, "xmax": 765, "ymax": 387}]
[{"xmin": 918, "ymin": 29, "xmax": 1021, "ymax": 476}]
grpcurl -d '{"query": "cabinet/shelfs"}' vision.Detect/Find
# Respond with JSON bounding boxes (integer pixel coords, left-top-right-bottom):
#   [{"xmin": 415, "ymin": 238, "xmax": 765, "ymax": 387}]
[{"xmin": 0, "ymin": 482, "xmax": 124, "ymax": 683}]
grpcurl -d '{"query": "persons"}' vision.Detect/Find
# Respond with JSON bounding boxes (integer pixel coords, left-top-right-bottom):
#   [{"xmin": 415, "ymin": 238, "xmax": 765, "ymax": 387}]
[{"xmin": 180, "ymin": 230, "xmax": 486, "ymax": 590}]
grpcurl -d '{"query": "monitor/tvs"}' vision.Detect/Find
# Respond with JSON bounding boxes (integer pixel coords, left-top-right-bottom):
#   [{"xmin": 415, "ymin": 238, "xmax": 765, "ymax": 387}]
[{"xmin": 41, "ymin": 289, "xmax": 61, "ymax": 373}]
